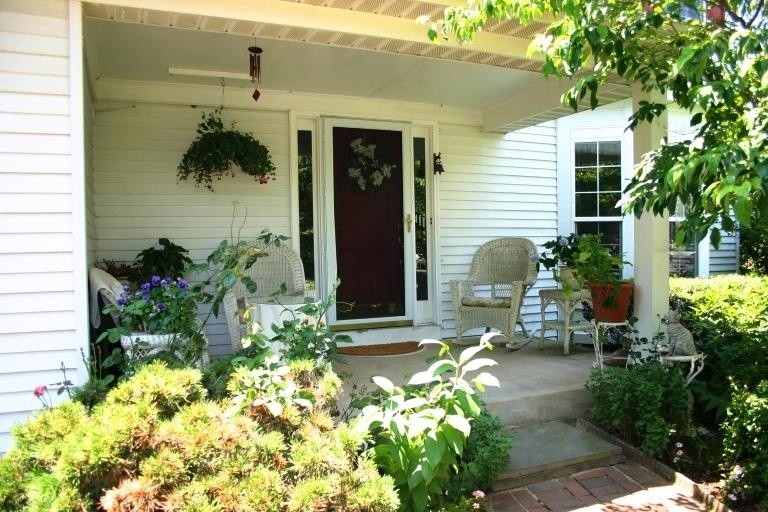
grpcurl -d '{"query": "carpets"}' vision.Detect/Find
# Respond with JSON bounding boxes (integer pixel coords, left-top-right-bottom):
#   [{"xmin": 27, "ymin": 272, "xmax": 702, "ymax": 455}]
[{"xmin": 335, "ymin": 341, "xmax": 424, "ymax": 356}]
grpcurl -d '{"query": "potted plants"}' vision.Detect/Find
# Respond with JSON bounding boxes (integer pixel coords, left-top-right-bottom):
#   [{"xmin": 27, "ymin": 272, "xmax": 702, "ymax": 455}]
[
  {"xmin": 176, "ymin": 106, "xmax": 277, "ymax": 191},
  {"xmin": 535, "ymin": 232, "xmax": 632, "ymax": 323}
]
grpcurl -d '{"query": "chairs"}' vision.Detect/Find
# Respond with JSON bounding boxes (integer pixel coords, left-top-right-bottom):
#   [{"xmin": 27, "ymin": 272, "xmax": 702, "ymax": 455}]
[
  {"xmin": 449, "ymin": 237, "xmax": 539, "ymax": 347},
  {"xmin": 221, "ymin": 245, "xmax": 307, "ymax": 353},
  {"xmin": 90, "ymin": 268, "xmax": 210, "ymax": 369}
]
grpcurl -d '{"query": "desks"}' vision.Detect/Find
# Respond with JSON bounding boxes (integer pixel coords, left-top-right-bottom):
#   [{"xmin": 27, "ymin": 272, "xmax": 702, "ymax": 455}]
[
  {"xmin": 541, "ymin": 290, "xmax": 596, "ymax": 355},
  {"xmin": 247, "ymin": 295, "xmax": 325, "ymax": 353}
]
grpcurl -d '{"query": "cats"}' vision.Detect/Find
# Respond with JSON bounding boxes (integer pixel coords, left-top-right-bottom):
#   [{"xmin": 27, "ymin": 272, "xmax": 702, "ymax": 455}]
[{"xmin": 664, "ymin": 307, "xmax": 697, "ymax": 356}]
